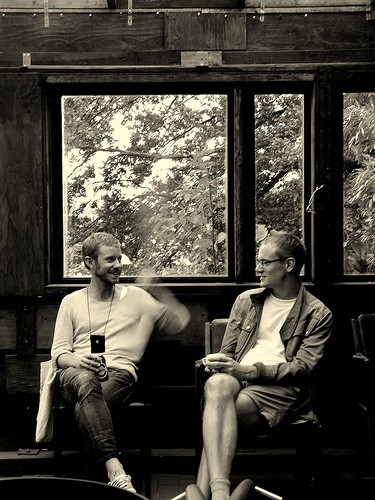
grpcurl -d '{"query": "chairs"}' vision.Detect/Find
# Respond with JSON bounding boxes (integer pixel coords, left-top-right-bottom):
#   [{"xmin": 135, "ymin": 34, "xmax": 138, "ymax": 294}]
[{"xmin": 51, "ymin": 313, "xmax": 375, "ymax": 500}]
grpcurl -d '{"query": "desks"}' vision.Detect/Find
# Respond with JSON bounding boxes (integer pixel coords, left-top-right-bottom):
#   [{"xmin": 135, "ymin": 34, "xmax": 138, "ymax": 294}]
[{"xmin": 0, "ymin": 477, "xmax": 150, "ymax": 500}]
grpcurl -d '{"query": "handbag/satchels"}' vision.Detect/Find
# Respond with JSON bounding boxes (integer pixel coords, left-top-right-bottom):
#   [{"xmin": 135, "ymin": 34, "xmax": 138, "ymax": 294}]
[{"xmin": 36, "ymin": 359, "xmax": 61, "ymax": 443}]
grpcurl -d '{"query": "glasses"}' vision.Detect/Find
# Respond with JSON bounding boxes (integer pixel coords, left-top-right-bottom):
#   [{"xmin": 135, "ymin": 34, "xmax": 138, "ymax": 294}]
[{"xmin": 255, "ymin": 256, "xmax": 293, "ymax": 266}]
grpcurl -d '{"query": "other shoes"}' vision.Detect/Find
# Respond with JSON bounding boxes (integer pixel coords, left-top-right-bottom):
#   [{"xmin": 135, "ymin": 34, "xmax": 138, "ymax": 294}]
[{"xmin": 107, "ymin": 474, "xmax": 137, "ymax": 494}]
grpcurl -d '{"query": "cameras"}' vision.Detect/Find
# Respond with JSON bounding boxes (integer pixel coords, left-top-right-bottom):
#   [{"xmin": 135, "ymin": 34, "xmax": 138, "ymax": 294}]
[{"xmin": 95, "ymin": 355, "xmax": 108, "ymax": 380}]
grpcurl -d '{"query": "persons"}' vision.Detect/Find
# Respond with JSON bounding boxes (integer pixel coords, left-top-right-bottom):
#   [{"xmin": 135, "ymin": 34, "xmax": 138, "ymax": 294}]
[
  {"xmin": 186, "ymin": 233, "xmax": 333, "ymax": 500},
  {"xmin": 51, "ymin": 232, "xmax": 191, "ymax": 493}
]
